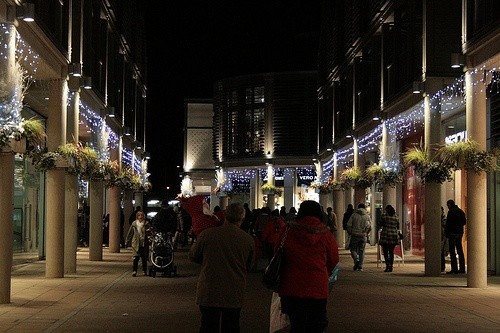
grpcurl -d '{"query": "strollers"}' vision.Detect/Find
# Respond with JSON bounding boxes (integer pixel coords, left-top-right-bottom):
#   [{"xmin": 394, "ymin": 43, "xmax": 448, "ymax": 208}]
[{"xmin": 148, "ymin": 230, "xmax": 178, "ymax": 277}]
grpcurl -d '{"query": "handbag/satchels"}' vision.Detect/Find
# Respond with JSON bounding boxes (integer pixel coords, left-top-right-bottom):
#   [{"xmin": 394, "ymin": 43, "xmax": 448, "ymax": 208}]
[
  {"xmin": 270, "ymin": 291, "xmax": 290, "ymax": 333},
  {"xmin": 262, "ymin": 226, "xmax": 291, "ymax": 292},
  {"xmin": 398, "ymin": 232, "xmax": 404, "ymax": 240}
]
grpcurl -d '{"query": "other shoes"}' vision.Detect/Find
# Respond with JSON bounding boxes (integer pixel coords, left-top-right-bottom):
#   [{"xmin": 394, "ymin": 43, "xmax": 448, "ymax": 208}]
[
  {"xmin": 447, "ymin": 270, "xmax": 465, "ymax": 274},
  {"xmin": 143, "ymin": 272, "xmax": 147, "ymax": 275},
  {"xmin": 384, "ymin": 263, "xmax": 393, "ymax": 272},
  {"xmin": 353, "ymin": 259, "xmax": 363, "ymax": 271},
  {"xmin": 133, "ymin": 272, "xmax": 137, "ymax": 277}
]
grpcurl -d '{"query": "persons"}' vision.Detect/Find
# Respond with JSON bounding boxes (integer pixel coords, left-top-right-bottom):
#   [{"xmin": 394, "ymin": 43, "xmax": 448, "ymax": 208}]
[
  {"xmin": 273, "ymin": 200, "xmax": 339, "ymax": 333},
  {"xmin": 255, "ymin": 208, "xmax": 270, "ymax": 229},
  {"xmin": 119, "ymin": 206, "xmax": 125, "ymax": 248},
  {"xmin": 243, "ymin": 202, "xmax": 252, "ymax": 232},
  {"xmin": 343, "ymin": 205, "xmax": 356, "ymax": 231},
  {"xmin": 440, "ymin": 206, "xmax": 450, "ymax": 272},
  {"xmin": 178, "ymin": 196, "xmax": 222, "ymax": 236},
  {"xmin": 129, "ymin": 210, "xmax": 155, "ymax": 276},
  {"xmin": 280, "ymin": 206, "xmax": 288, "ymax": 221},
  {"xmin": 347, "ymin": 204, "xmax": 372, "ymax": 271},
  {"xmin": 262, "ymin": 211, "xmax": 287, "ymax": 247},
  {"xmin": 325, "ymin": 207, "xmax": 338, "ymax": 232},
  {"xmin": 187, "ymin": 204, "xmax": 258, "ymax": 333},
  {"xmin": 286, "ymin": 206, "xmax": 297, "ymax": 223},
  {"xmin": 446, "ymin": 199, "xmax": 468, "ymax": 274},
  {"xmin": 103, "ymin": 213, "xmax": 110, "ymax": 248},
  {"xmin": 152, "ymin": 199, "xmax": 180, "ymax": 246},
  {"xmin": 212, "ymin": 206, "xmax": 224, "ymax": 224},
  {"xmin": 375, "ymin": 204, "xmax": 403, "ymax": 272}
]
